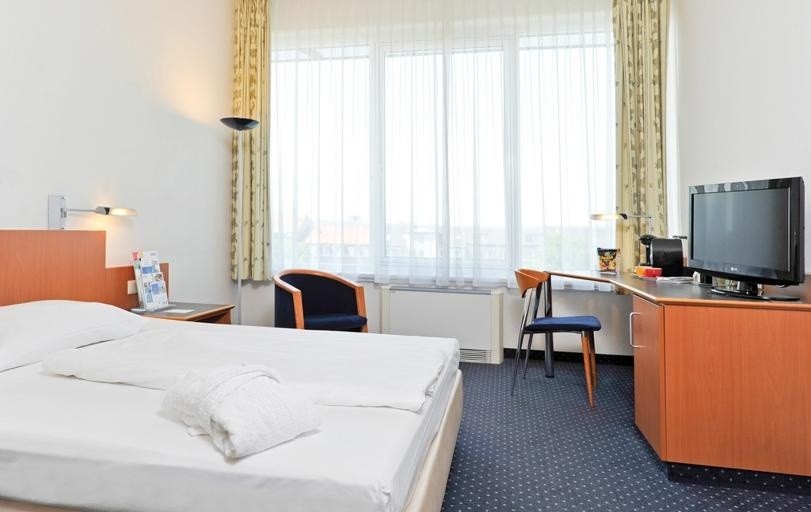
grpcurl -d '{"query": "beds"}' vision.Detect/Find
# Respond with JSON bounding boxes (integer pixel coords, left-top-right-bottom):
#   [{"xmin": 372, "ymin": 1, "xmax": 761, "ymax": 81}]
[{"xmin": 0, "ymin": 226, "xmax": 464, "ymax": 512}]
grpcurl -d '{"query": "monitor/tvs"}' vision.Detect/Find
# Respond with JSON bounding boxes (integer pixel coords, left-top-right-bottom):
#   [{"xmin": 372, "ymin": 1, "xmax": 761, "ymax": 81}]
[{"xmin": 686, "ymin": 176, "xmax": 806, "ymax": 301}]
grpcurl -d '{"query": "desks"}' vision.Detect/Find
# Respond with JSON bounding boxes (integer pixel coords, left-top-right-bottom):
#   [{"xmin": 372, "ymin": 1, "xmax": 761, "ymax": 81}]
[
  {"xmin": 129, "ymin": 303, "xmax": 236, "ymax": 326},
  {"xmin": 543, "ymin": 267, "xmax": 810, "ymax": 483}
]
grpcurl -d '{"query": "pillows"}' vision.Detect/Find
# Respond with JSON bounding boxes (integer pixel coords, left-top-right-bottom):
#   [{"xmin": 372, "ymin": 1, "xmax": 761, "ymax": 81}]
[{"xmin": 1, "ymin": 297, "xmax": 228, "ymax": 397}]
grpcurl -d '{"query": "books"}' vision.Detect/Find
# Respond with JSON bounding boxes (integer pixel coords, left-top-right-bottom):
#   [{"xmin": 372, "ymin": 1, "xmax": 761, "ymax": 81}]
[{"xmin": 131, "ymin": 248, "xmax": 169, "ymax": 311}]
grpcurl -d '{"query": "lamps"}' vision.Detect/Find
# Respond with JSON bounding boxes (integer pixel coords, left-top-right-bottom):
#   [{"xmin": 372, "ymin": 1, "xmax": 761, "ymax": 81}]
[
  {"xmin": 590, "ymin": 209, "xmax": 652, "ymax": 254},
  {"xmin": 220, "ymin": 3, "xmax": 259, "ymax": 133},
  {"xmin": 49, "ymin": 196, "xmax": 136, "ymax": 226}
]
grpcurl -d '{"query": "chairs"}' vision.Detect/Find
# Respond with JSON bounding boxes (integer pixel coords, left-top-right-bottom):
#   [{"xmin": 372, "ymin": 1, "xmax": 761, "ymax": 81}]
[
  {"xmin": 509, "ymin": 266, "xmax": 605, "ymax": 409},
  {"xmin": 271, "ymin": 267, "xmax": 369, "ymax": 335}
]
